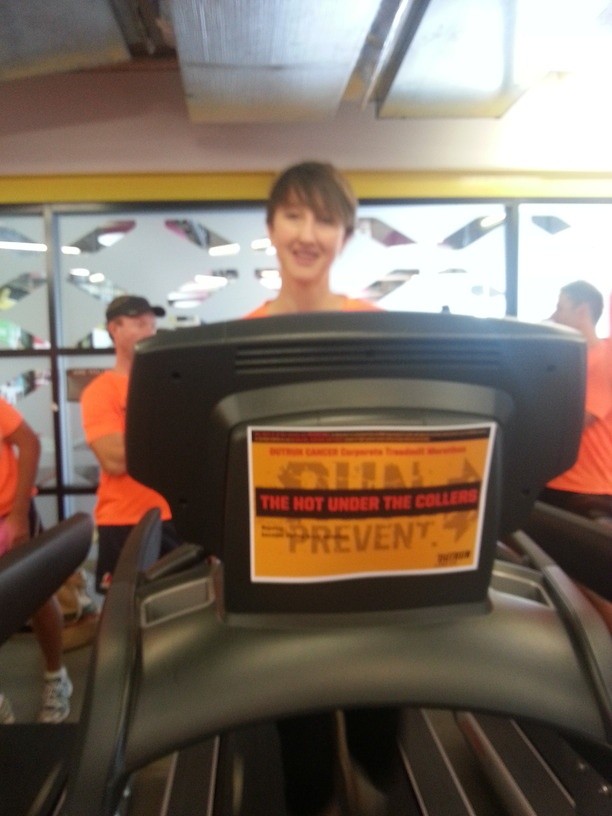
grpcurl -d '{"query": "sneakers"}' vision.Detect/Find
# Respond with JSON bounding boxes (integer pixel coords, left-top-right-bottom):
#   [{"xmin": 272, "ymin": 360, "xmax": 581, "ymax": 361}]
[{"xmin": 39, "ymin": 673, "xmax": 74, "ymax": 723}]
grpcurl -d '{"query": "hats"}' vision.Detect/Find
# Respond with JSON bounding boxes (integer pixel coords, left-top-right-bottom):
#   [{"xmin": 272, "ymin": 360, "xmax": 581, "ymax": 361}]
[{"xmin": 106, "ymin": 297, "xmax": 165, "ymax": 320}]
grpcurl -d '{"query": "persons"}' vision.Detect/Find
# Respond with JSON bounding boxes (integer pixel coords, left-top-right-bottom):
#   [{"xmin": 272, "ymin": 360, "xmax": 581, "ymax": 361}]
[
  {"xmin": 79, "ymin": 293, "xmax": 186, "ymax": 596},
  {"xmin": 229, "ymin": 160, "xmax": 388, "ymax": 320},
  {"xmin": 2, "ymin": 398, "xmax": 79, "ymax": 723},
  {"xmin": 535, "ymin": 281, "xmax": 611, "ymax": 527}
]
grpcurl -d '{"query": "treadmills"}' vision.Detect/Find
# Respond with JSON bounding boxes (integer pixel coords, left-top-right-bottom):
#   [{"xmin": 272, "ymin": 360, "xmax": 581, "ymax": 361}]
[
  {"xmin": 1, "ymin": 510, "xmax": 99, "ymax": 815},
  {"xmin": 24, "ymin": 317, "xmax": 612, "ymax": 815}
]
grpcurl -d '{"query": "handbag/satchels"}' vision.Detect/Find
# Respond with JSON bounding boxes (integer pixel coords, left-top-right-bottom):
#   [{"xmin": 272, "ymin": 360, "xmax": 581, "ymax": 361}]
[{"xmin": 26, "ymin": 569, "xmax": 101, "ymax": 656}]
